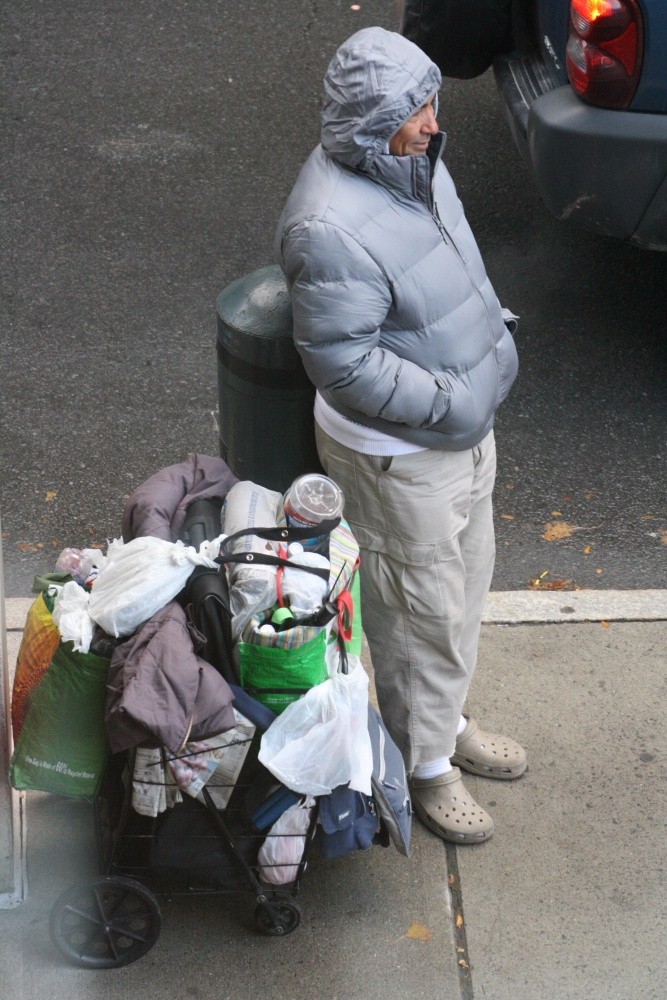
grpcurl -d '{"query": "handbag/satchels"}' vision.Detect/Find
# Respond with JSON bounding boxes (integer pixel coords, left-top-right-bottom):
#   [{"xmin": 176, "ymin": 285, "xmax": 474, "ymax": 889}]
[
  {"xmin": 185, "ymin": 493, "xmax": 418, "ymax": 861},
  {"xmin": 10, "ymin": 590, "xmax": 116, "ymax": 798}
]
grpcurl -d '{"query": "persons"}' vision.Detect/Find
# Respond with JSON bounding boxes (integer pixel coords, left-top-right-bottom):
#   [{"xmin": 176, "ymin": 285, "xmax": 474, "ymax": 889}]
[{"xmin": 276, "ymin": 26, "xmax": 529, "ymax": 843}]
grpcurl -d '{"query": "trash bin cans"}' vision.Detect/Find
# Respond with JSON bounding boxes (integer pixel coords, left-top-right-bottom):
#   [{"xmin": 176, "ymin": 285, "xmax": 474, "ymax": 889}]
[{"xmin": 216, "ymin": 266, "xmax": 328, "ymax": 495}]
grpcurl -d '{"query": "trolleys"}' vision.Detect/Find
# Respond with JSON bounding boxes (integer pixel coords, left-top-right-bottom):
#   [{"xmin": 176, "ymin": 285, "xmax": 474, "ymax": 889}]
[{"xmin": 48, "ymin": 734, "xmax": 321, "ymax": 970}]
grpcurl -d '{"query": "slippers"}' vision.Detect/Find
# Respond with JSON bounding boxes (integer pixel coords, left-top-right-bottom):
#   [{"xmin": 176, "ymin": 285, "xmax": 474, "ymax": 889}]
[
  {"xmin": 407, "ymin": 764, "xmax": 495, "ymax": 844},
  {"xmin": 451, "ymin": 713, "xmax": 528, "ymax": 778}
]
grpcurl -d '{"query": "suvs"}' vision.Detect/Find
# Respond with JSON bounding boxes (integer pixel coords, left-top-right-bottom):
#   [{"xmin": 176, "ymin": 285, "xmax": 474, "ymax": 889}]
[{"xmin": 403, "ymin": 0, "xmax": 667, "ymax": 253}]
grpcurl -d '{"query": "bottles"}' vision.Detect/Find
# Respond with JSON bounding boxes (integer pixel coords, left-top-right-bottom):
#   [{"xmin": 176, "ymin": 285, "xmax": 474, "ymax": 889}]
[{"xmin": 277, "ymin": 472, "xmax": 345, "ymax": 552}]
